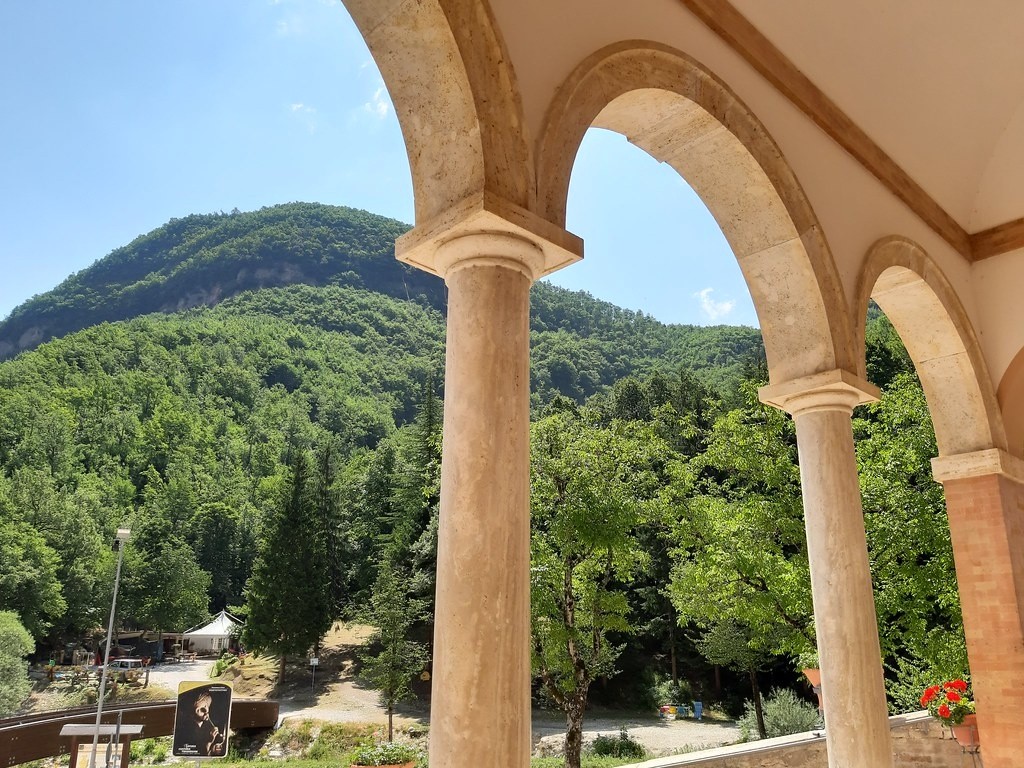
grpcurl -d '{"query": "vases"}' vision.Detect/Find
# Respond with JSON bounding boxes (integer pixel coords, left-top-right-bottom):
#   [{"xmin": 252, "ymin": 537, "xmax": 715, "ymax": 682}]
[{"xmin": 952, "ymin": 714, "xmax": 980, "ymax": 747}]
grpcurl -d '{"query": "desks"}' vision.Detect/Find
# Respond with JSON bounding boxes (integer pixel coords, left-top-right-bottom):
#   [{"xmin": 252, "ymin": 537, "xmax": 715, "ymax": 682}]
[{"xmin": 178, "ymin": 654, "xmax": 195, "ymax": 663}]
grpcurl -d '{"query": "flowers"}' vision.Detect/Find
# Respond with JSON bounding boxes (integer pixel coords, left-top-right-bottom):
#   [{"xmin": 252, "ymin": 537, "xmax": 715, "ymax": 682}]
[{"xmin": 920, "ymin": 679, "xmax": 977, "ymax": 727}]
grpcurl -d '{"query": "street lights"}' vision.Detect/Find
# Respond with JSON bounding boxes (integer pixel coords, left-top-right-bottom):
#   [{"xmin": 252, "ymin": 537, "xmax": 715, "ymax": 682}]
[{"xmin": 89, "ymin": 528, "xmax": 131, "ymax": 768}]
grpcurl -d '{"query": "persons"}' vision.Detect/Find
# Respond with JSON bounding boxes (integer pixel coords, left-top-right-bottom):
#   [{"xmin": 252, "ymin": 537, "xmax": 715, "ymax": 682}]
[{"xmin": 174, "ymin": 690, "xmax": 223, "ymax": 755}]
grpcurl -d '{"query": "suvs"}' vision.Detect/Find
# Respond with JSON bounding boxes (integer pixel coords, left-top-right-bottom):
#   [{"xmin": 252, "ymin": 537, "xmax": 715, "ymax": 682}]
[{"xmin": 97, "ymin": 659, "xmax": 143, "ymax": 678}]
